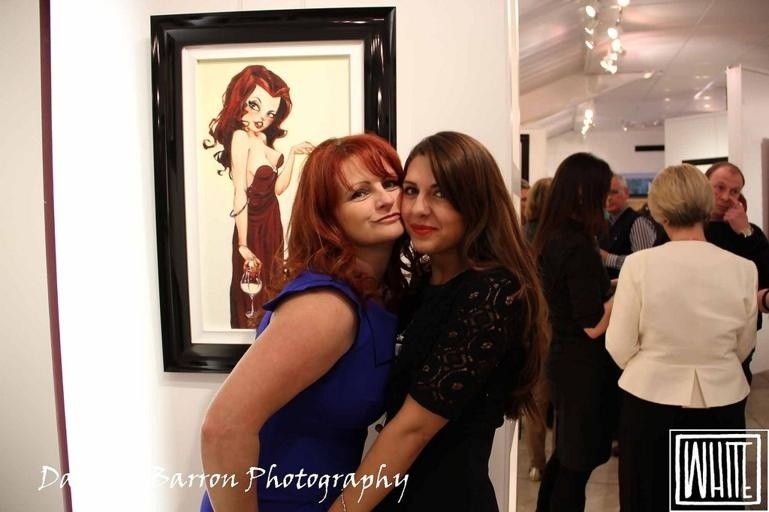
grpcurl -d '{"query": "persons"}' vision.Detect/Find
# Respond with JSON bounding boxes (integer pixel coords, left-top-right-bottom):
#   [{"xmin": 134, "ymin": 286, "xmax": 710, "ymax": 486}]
[
  {"xmin": 203, "ymin": 65, "xmax": 318, "ymax": 329},
  {"xmin": 325, "ymin": 131, "xmax": 548, "ymax": 512},
  {"xmin": 199, "ymin": 134, "xmax": 413, "ymax": 512},
  {"xmin": 521, "ymin": 151, "xmax": 769, "ymax": 512}
]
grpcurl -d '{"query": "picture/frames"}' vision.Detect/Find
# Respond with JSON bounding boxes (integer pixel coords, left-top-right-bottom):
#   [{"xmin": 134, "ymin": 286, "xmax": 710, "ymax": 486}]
[{"xmin": 151, "ymin": 6, "xmax": 397, "ymax": 373}]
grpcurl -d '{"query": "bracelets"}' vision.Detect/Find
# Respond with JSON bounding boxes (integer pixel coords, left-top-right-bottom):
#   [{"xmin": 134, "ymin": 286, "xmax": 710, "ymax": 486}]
[{"xmin": 341, "ymin": 492, "xmax": 347, "ymax": 512}]
